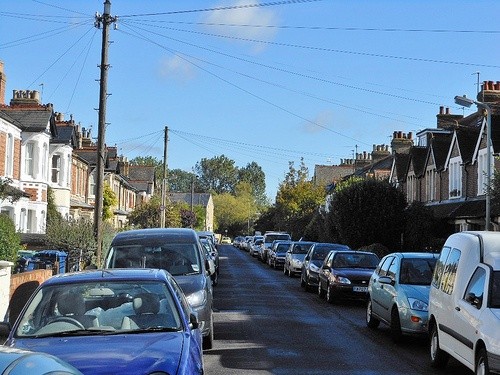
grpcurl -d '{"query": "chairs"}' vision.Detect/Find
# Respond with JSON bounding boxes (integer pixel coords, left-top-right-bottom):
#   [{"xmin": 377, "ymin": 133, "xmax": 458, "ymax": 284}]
[
  {"xmin": 120, "ymin": 294, "xmax": 174, "ymax": 328},
  {"xmin": 53, "ymin": 294, "xmax": 99, "ymax": 329},
  {"xmin": 295, "ymin": 246, "xmax": 301, "ymax": 251},
  {"xmin": 336, "ymin": 257, "xmax": 345, "ymax": 266},
  {"xmin": 316, "ymin": 248, "xmax": 325, "ymax": 259}
]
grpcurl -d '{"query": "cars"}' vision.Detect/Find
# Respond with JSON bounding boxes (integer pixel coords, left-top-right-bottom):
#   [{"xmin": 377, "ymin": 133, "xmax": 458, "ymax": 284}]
[
  {"xmin": 0, "ymin": 345, "xmax": 83, "ymax": 375},
  {"xmin": 301, "ymin": 243, "xmax": 351, "ymax": 292},
  {"xmin": 0, "ymin": 270, "xmax": 207, "ymax": 375},
  {"xmin": 364, "ymin": 252, "xmax": 442, "ymax": 349},
  {"xmin": 316, "ymin": 250, "xmax": 380, "ymax": 304},
  {"xmin": 233, "ymin": 230, "xmax": 297, "ymax": 269},
  {"xmin": 221, "ymin": 236, "xmax": 232, "ymax": 244},
  {"xmin": 283, "ymin": 241, "xmax": 318, "ymax": 277},
  {"xmin": 197, "ymin": 230, "xmax": 220, "ymax": 286}
]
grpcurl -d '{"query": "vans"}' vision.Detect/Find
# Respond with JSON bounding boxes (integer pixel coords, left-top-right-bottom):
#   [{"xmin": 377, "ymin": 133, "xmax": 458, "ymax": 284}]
[
  {"xmin": 102, "ymin": 228, "xmax": 218, "ymax": 350},
  {"xmin": 426, "ymin": 231, "xmax": 500, "ymax": 375}
]
